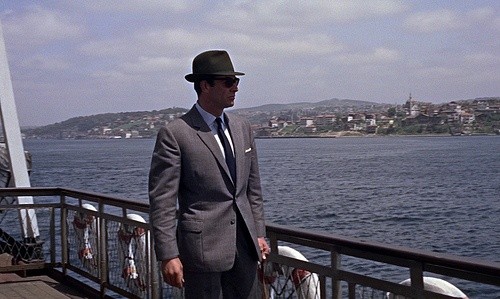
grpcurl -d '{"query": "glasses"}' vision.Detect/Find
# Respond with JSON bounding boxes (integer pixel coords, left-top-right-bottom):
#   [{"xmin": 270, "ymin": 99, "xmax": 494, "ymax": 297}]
[{"xmin": 208, "ymin": 76, "xmax": 240, "ymax": 88}]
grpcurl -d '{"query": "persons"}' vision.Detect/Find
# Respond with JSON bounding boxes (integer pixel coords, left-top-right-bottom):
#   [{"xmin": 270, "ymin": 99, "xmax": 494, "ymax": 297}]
[{"xmin": 147, "ymin": 50, "xmax": 268, "ymax": 299}]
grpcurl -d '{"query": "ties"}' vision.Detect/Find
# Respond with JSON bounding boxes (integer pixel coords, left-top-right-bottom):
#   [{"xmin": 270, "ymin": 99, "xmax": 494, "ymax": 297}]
[{"xmin": 215, "ymin": 117, "xmax": 236, "ymax": 186}]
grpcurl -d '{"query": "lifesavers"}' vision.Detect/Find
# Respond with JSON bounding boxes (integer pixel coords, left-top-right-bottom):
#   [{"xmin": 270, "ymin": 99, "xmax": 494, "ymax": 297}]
[
  {"xmin": 389, "ymin": 275, "xmax": 468, "ymax": 299},
  {"xmin": 117, "ymin": 214, "xmax": 155, "ymax": 294},
  {"xmin": 255, "ymin": 245, "xmax": 321, "ymax": 299},
  {"xmin": 72, "ymin": 203, "xmax": 100, "ymax": 275}
]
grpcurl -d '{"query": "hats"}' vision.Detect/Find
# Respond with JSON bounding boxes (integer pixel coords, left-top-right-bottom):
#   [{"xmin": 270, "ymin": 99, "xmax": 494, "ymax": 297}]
[{"xmin": 184, "ymin": 50, "xmax": 246, "ymax": 83}]
